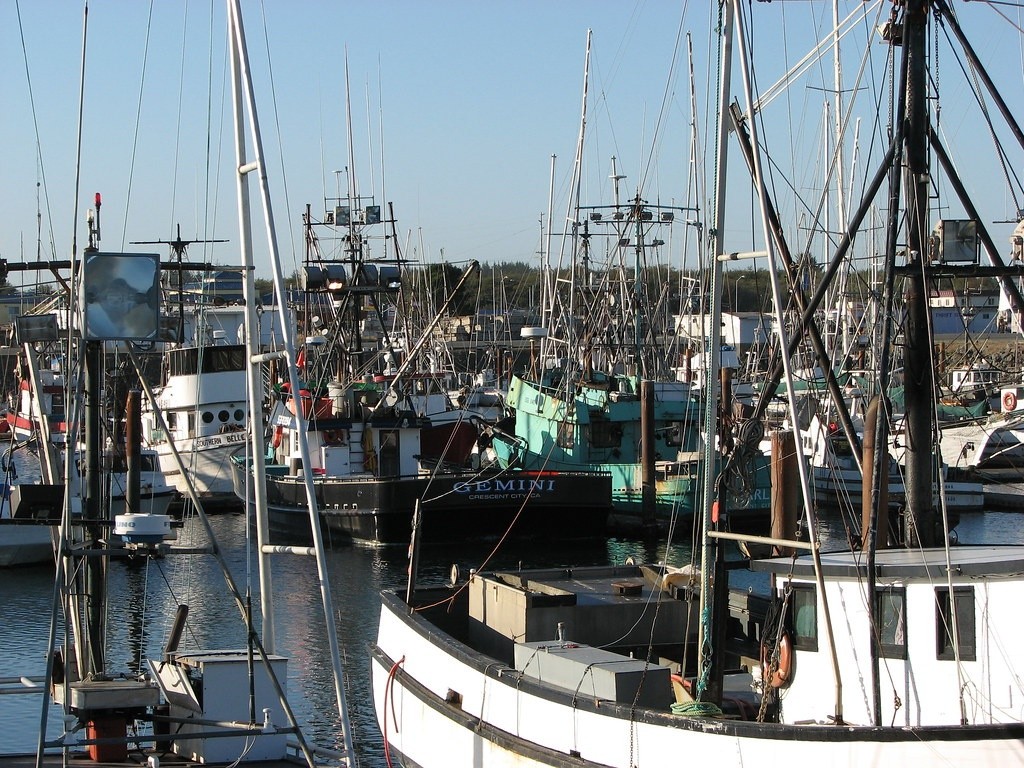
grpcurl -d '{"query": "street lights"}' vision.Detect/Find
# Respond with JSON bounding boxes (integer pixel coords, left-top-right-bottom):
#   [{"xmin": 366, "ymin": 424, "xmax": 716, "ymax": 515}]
[
  {"xmin": 500, "ymin": 275, "xmax": 508, "ymax": 316},
  {"xmin": 735, "ymin": 276, "xmax": 746, "ymax": 312}
]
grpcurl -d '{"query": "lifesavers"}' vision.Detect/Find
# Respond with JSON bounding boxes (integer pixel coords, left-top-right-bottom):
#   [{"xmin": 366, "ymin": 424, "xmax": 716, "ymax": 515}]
[
  {"xmin": 273, "ymin": 426, "xmax": 282, "ymax": 448},
  {"xmin": 1002, "ymin": 392, "xmax": 1017, "ymax": 410},
  {"xmin": 763, "ymin": 633, "xmax": 792, "ymax": 688}
]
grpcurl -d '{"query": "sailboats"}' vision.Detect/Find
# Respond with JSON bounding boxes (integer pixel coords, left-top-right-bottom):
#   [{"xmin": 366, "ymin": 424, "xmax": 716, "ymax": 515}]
[{"xmin": 0, "ymin": 1, "xmax": 1024, "ymax": 768}]
